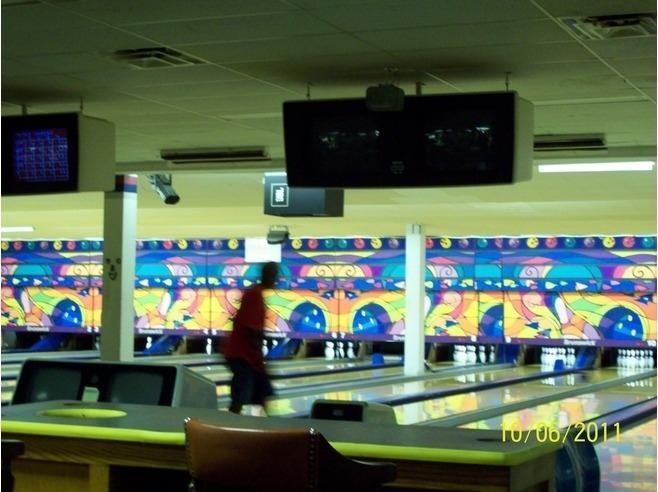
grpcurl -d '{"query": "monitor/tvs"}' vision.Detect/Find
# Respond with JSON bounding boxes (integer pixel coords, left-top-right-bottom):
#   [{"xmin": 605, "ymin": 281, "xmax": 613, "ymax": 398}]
[
  {"xmin": 12, "ymin": 360, "xmax": 217, "ymax": 408},
  {"xmin": 1, "ymin": 116, "xmax": 115, "ymax": 194},
  {"xmin": 284, "ymin": 91, "xmax": 535, "ymax": 190}
]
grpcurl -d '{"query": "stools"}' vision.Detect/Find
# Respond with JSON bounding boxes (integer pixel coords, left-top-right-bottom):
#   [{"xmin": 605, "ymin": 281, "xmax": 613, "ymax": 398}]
[{"xmin": 183, "ymin": 417, "xmax": 399, "ymax": 491}]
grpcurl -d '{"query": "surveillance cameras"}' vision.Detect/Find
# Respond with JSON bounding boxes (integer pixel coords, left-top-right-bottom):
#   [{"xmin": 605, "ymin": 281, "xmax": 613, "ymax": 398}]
[{"xmin": 148, "ymin": 174, "xmax": 177, "ymax": 204}]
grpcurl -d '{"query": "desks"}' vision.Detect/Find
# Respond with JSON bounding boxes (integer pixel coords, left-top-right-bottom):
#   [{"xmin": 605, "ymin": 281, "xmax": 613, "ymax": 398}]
[{"xmin": 0, "ymin": 397, "xmax": 565, "ymax": 490}]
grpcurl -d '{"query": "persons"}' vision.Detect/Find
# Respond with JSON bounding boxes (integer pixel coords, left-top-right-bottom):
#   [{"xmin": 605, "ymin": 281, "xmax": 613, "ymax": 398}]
[{"xmin": 223, "ymin": 260, "xmax": 279, "ymax": 418}]
